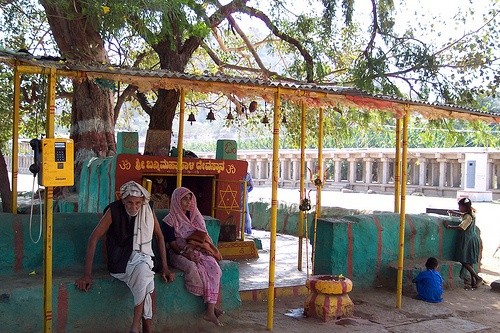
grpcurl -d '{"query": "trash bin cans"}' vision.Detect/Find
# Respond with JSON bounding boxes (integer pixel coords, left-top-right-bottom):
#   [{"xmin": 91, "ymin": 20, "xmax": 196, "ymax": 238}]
[{"xmin": 304, "ymin": 275, "xmax": 355, "ymax": 321}]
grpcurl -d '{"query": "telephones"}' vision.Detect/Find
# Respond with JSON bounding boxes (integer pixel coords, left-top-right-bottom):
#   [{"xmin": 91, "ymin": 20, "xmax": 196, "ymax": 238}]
[{"xmin": 30, "ymin": 139, "xmax": 75, "ymax": 186}]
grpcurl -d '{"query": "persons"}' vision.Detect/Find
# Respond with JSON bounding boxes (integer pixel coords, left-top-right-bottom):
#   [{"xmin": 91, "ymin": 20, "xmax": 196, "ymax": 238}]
[
  {"xmin": 74, "ymin": 181, "xmax": 176, "ymax": 333},
  {"xmin": 240, "ymin": 172, "xmax": 253, "ymax": 234},
  {"xmin": 412, "ymin": 257, "xmax": 443, "ymax": 303},
  {"xmin": 161, "ymin": 188, "xmax": 225, "ymax": 327},
  {"xmin": 443, "ymin": 198, "xmax": 483, "ymax": 290}
]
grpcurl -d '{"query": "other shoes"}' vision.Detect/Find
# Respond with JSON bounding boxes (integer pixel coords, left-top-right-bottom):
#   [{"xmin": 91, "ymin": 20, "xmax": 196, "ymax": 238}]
[{"xmin": 473, "ymin": 275, "xmax": 483, "ymax": 287}]
